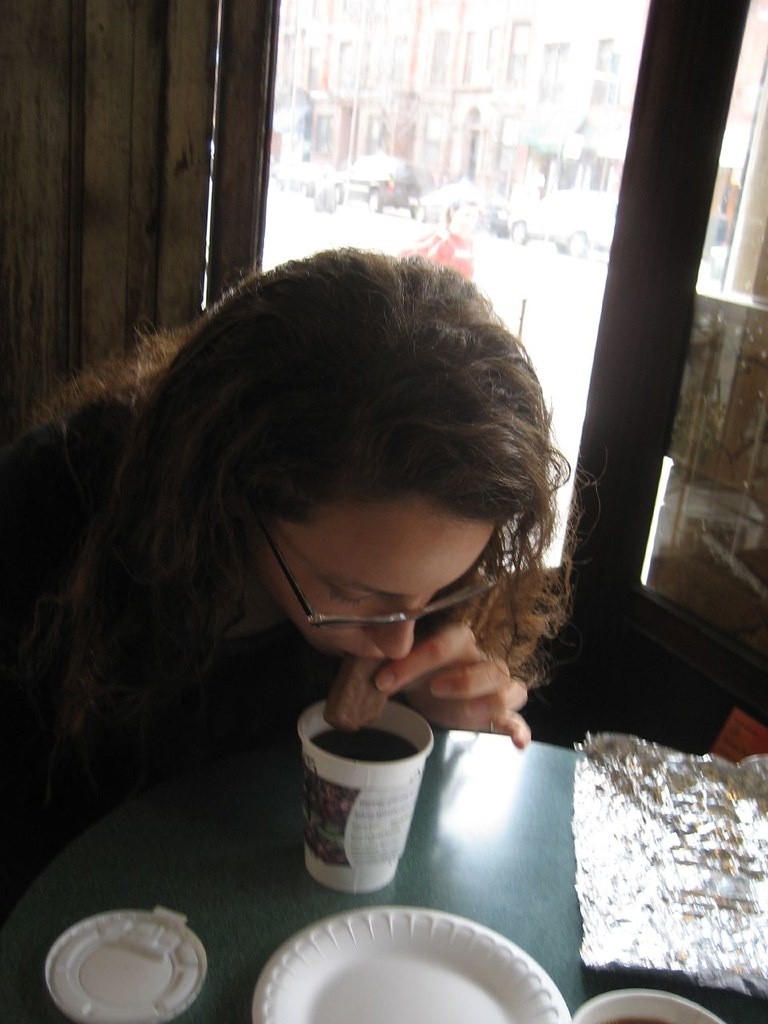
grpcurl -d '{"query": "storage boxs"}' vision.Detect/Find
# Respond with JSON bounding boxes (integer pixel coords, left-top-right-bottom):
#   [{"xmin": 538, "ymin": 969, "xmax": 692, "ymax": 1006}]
[{"xmin": 646, "ymin": 287, "xmax": 767, "ymax": 660}]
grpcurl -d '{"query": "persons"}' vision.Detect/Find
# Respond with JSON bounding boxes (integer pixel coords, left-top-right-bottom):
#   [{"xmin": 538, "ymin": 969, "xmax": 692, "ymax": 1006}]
[
  {"xmin": 400, "ymin": 199, "xmax": 480, "ymax": 286},
  {"xmin": 1, "ymin": 244, "xmax": 572, "ymax": 920}
]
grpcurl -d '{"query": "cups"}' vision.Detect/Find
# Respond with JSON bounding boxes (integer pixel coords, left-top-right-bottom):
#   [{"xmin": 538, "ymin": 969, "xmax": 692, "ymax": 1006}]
[
  {"xmin": 573, "ymin": 989, "xmax": 727, "ymax": 1024},
  {"xmin": 297, "ymin": 700, "xmax": 434, "ymax": 895}
]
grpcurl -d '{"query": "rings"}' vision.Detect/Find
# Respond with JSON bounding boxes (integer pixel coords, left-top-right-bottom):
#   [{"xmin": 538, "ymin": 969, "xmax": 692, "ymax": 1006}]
[{"xmin": 489, "ymin": 718, "xmax": 493, "ymax": 733}]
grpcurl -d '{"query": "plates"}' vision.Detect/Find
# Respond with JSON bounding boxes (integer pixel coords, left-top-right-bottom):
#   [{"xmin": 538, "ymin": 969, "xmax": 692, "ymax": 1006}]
[{"xmin": 253, "ymin": 905, "xmax": 572, "ymax": 1024}]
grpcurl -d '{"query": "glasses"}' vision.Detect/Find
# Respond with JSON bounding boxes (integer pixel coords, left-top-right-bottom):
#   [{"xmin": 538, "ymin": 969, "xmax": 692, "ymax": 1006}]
[{"xmin": 243, "ymin": 488, "xmax": 498, "ymax": 630}]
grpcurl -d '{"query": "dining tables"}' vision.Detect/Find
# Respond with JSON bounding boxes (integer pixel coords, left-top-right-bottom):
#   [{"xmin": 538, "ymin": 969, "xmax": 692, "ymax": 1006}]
[{"xmin": 2, "ymin": 727, "xmax": 766, "ymax": 1024}]
